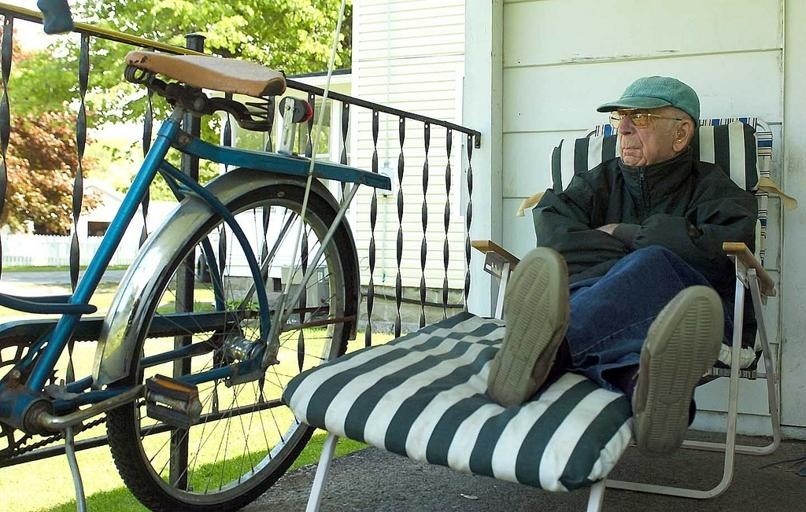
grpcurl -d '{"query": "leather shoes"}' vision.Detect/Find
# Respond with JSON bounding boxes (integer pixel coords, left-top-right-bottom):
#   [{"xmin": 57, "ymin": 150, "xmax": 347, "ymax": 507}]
[
  {"xmin": 487, "ymin": 246, "xmax": 570, "ymax": 407},
  {"xmin": 631, "ymin": 286, "xmax": 724, "ymax": 457}
]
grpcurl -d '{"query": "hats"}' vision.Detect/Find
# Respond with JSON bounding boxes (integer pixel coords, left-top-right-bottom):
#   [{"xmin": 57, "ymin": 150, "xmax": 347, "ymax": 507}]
[{"xmin": 597, "ymin": 75, "xmax": 700, "ymax": 127}]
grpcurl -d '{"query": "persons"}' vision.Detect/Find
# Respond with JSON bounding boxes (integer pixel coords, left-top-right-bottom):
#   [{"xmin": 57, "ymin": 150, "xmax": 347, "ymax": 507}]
[{"xmin": 482, "ymin": 74, "xmax": 761, "ymax": 460}]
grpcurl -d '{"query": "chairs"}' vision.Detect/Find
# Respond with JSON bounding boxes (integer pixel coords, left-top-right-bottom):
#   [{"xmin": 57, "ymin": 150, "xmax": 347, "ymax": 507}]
[{"xmin": 280, "ymin": 118, "xmax": 780, "ymax": 512}]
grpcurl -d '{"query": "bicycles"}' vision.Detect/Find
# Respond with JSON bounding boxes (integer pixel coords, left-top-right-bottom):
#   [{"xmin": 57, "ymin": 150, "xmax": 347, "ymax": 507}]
[{"xmin": 1, "ymin": 0, "xmax": 393, "ymax": 510}]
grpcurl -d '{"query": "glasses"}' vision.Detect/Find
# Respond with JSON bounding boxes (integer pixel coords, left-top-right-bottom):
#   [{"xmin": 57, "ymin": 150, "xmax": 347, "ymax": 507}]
[{"xmin": 609, "ymin": 109, "xmax": 683, "ymax": 129}]
